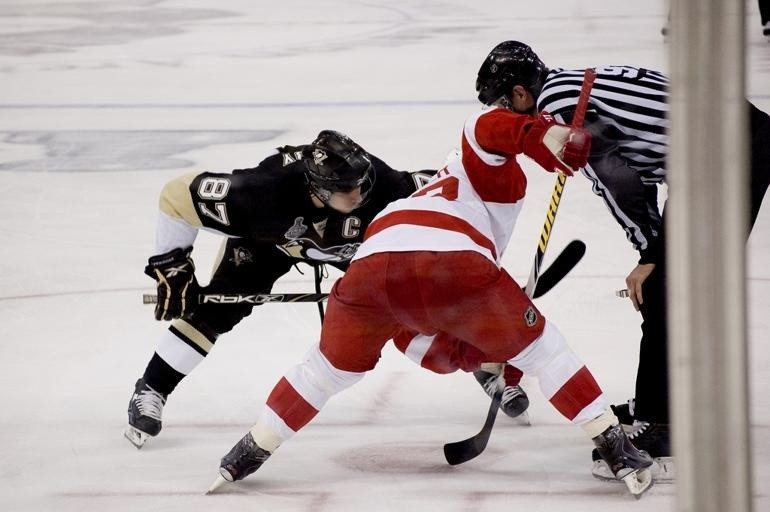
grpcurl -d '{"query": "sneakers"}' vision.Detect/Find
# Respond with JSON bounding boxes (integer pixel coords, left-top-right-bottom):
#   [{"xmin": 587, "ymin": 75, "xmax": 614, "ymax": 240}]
[
  {"xmin": 128, "ymin": 378, "xmax": 166, "ymax": 436},
  {"xmin": 592, "ymin": 398, "xmax": 672, "ymax": 480},
  {"xmin": 220, "ymin": 431, "xmax": 272, "ymax": 482},
  {"xmin": 473, "ymin": 370, "xmax": 529, "ymax": 417}
]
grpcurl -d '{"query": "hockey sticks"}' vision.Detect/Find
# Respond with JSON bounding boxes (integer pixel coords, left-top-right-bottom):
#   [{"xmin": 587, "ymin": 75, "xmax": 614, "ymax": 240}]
[
  {"xmin": 443, "ymin": 71, "xmax": 596, "ymax": 465},
  {"xmin": 143, "ymin": 238, "xmax": 585, "ymax": 301}
]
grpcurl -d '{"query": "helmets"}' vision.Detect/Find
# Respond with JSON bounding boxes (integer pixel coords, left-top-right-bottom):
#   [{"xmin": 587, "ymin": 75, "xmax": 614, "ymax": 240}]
[
  {"xmin": 477, "ymin": 40, "xmax": 545, "ymax": 112},
  {"xmin": 301, "ymin": 130, "xmax": 376, "ymax": 210}
]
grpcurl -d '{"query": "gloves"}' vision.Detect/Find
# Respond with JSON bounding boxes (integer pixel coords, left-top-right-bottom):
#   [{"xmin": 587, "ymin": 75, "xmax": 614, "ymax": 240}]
[
  {"xmin": 521, "ymin": 111, "xmax": 593, "ymax": 177},
  {"xmin": 145, "ymin": 244, "xmax": 198, "ymax": 320}
]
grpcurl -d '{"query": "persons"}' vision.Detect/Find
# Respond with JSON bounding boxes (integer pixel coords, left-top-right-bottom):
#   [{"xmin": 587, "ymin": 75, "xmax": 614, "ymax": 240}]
[
  {"xmin": 219, "ymin": 105, "xmax": 653, "ymax": 485},
  {"xmin": 126, "ymin": 127, "xmax": 530, "ymax": 438},
  {"xmin": 472, "ymin": 38, "xmax": 770, "ymax": 462}
]
